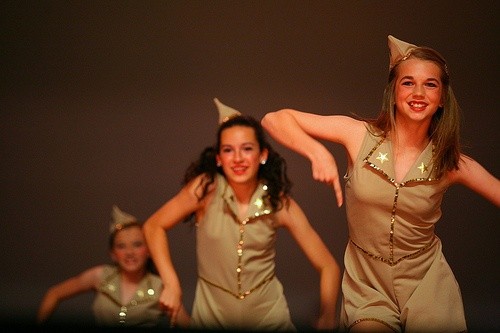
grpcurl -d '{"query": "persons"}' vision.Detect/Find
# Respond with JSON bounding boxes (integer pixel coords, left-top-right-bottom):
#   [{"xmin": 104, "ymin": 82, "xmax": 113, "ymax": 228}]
[
  {"xmin": 261, "ymin": 35, "xmax": 500, "ymax": 333},
  {"xmin": 143, "ymin": 98, "xmax": 341, "ymax": 333},
  {"xmin": 38, "ymin": 205, "xmax": 191, "ymax": 333}
]
